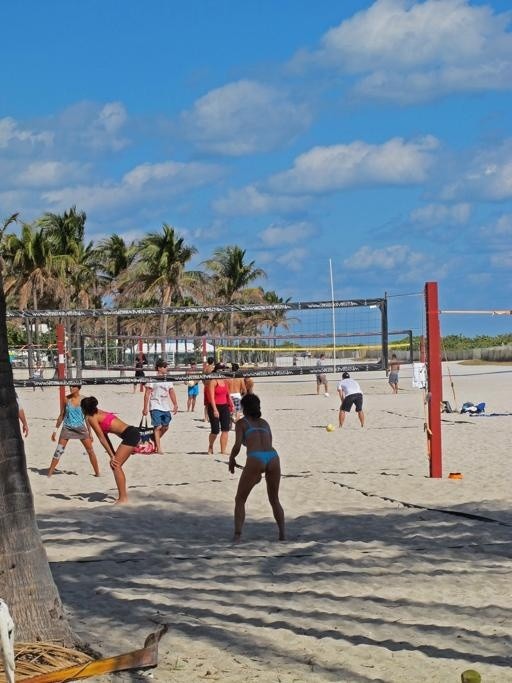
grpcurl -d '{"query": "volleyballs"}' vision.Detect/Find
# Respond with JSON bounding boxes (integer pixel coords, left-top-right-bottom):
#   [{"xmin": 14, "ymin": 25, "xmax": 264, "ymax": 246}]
[
  {"xmin": 188, "ymin": 380, "xmax": 194, "ymax": 387},
  {"xmin": 327, "ymin": 424, "xmax": 335, "ymax": 432}
]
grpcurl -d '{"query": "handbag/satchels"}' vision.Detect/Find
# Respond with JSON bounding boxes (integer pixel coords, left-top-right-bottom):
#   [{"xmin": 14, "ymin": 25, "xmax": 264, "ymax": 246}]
[{"xmin": 132, "ymin": 424, "xmax": 156, "ymax": 454}]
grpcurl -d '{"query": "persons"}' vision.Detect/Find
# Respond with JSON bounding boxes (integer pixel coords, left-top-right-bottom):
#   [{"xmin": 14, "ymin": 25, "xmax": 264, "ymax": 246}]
[
  {"xmin": 336, "ymin": 371, "xmax": 365, "ymax": 428},
  {"xmin": 227, "ymin": 393, "xmax": 285, "ymax": 542},
  {"xmin": 387, "ymin": 353, "xmax": 400, "ymax": 393},
  {"xmin": 292, "ymin": 353, "xmax": 299, "ymax": 366},
  {"xmin": 183, "ymin": 356, "xmax": 255, "ymax": 431},
  {"xmin": 205, "ymin": 361, "xmax": 236, "ymax": 456},
  {"xmin": 132, "ymin": 353, "xmax": 148, "ymax": 393},
  {"xmin": 47, "ymin": 384, "xmax": 99, "ymax": 476},
  {"xmin": 142, "ymin": 358, "xmax": 179, "ymax": 453},
  {"xmin": 80, "ymin": 395, "xmax": 141, "ymax": 505},
  {"xmin": 15, "ymin": 390, "xmax": 30, "ymax": 438},
  {"xmin": 32, "ymin": 359, "xmax": 46, "ymax": 392},
  {"xmin": 316, "ymin": 353, "xmax": 329, "ymax": 396}
]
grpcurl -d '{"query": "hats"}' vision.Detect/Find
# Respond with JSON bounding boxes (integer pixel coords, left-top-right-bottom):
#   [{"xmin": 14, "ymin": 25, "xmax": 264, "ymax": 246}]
[
  {"xmin": 214, "ymin": 362, "xmax": 230, "ymax": 370},
  {"xmin": 156, "ymin": 358, "xmax": 169, "ymax": 367}
]
